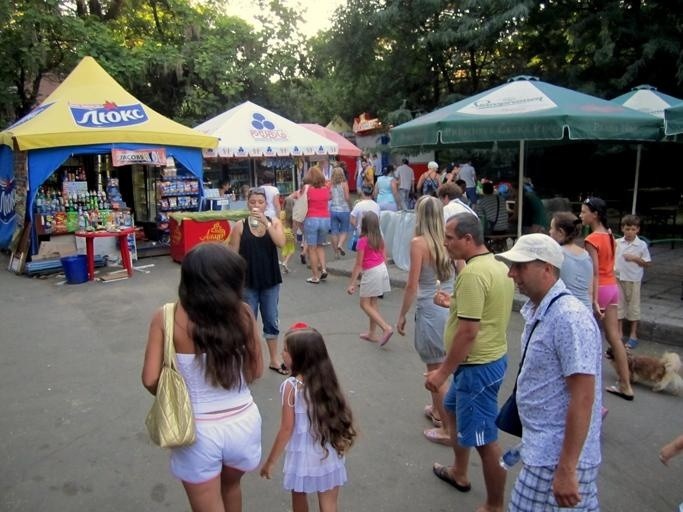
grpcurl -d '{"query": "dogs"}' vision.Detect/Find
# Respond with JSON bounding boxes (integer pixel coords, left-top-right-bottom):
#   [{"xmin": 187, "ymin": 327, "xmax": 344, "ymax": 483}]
[{"xmin": 605, "ymin": 342, "xmax": 683, "ymax": 396}]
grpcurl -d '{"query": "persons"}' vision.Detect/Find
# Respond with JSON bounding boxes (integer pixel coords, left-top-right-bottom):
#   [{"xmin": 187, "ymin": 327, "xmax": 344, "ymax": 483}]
[
  {"xmin": 251, "ymin": 322, "xmax": 358, "ymax": 511},
  {"xmin": 360, "ymin": 156, "xmax": 415, "ymax": 212},
  {"xmin": 261, "ymin": 171, "xmax": 282, "ymax": 221},
  {"xmin": 491, "ymin": 230, "xmax": 606, "ymax": 512},
  {"xmin": 416, "ymin": 154, "xmax": 653, "ymax": 401},
  {"xmin": 393, "ymin": 195, "xmax": 465, "ymax": 447},
  {"xmin": 230, "ymin": 188, "xmax": 290, "ymax": 375},
  {"xmin": 278, "ymin": 222, "xmax": 296, "ymax": 273},
  {"xmin": 216, "ymin": 176, "xmax": 233, "ymax": 200},
  {"xmin": 347, "ymin": 210, "xmax": 392, "ymax": 346},
  {"xmin": 138, "ymin": 240, "xmax": 265, "ymax": 512},
  {"xmin": 239, "ymin": 184, "xmax": 250, "ymax": 201},
  {"xmin": 349, "ymin": 183, "xmax": 380, "ymax": 228},
  {"xmin": 421, "ymin": 212, "xmax": 516, "ymax": 512},
  {"xmin": 329, "ymin": 167, "xmax": 350, "ymax": 258},
  {"xmin": 656, "ymin": 431, "xmax": 682, "ymax": 467},
  {"xmin": 285, "ymin": 166, "xmax": 333, "ymax": 284}
]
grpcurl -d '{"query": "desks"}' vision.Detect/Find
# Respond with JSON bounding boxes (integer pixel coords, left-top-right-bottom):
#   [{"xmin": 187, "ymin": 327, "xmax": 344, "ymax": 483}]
[{"xmin": 75, "ymin": 227, "xmax": 136, "ymax": 282}]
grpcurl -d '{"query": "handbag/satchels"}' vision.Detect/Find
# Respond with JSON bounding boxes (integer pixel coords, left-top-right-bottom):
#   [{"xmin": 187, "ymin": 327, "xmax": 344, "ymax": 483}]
[
  {"xmin": 290, "ymin": 194, "xmax": 308, "ymax": 223},
  {"xmin": 494, "ymin": 392, "xmax": 522, "ymax": 437},
  {"xmin": 485, "ymin": 221, "xmax": 496, "ymax": 234},
  {"xmin": 144, "ymin": 367, "xmax": 195, "ymax": 449},
  {"xmin": 348, "ymin": 226, "xmax": 358, "ymax": 251}
]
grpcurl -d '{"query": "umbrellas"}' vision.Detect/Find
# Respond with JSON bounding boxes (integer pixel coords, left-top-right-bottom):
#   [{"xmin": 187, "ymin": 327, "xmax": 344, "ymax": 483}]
[
  {"xmin": 388, "ymin": 75, "xmax": 665, "ymax": 243},
  {"xmin": 601, "ymin": 85, "xmax": 682, "ymax": 215}
]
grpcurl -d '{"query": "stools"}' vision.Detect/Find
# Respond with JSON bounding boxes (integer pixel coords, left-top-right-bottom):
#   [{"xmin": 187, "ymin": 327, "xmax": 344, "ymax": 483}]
[{"xmin": 481, "ymin": 233, "xmax": 520, "ymax": 253}]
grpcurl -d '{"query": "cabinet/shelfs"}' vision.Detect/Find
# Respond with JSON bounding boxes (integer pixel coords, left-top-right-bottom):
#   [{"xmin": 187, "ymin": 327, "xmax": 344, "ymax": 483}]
[{"xmin": 154, "ymin": 176, "xmax": 202, "ymax": 249}]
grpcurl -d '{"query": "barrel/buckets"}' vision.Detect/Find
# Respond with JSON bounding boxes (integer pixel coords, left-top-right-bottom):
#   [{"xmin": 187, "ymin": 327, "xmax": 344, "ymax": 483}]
[{"xmin": 60, "ymin": 254, "xmax": 89, "ymax": 284}]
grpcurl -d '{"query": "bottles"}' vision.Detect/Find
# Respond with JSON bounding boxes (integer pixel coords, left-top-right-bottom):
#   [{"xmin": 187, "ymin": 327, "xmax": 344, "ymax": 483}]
[
  {"xmin": 62, "ymin": 168, "xmax": 86, "ymax": 181},
  {"xmin": 500, "ymin": 442, "xmax": 524, "ymax": 470},
  {"xmin": 576, "ymin": 191, "xmax": 593, "ymax": 203},
  {"xmin": 250, "ymin": 205, "xmax": 262, "ymax": 230},
  {"xmin": 35, "ymin": 187, "xmax": 131, "ymax": 231}
]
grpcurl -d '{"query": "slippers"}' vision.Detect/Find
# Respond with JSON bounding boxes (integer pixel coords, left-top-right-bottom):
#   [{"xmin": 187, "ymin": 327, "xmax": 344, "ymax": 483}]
[
  {"xmin": 424, "ymin": 428, "xmax": 452, "ymax": 446},
  {"xmin": 269, "ymin": 363, "xmax": 290, "ymax": 375},
  {"xmin": 423, "ymin": 403, "xmax": 442, "ymax": 426},
  {"xmin": 358, "ymin": 331, "xmax": 378, "ymax": 343},
  {"xmin": 432, "ymin": 463, "xmax": 471, "ymax": 490},
  {"xmin": 378, "ymin": 329, "xmax": 394, "ymax": 346},
  {"xmin": 624, "ymin": 338, "xmax": 639, "ymax": 348},
  {"xmin": 605, "ymin": 385, "xmax": 634, "ymax": 400}
]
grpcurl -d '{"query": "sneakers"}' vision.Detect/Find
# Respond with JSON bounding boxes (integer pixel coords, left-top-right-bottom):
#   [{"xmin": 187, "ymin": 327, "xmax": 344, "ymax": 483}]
[{"xmin": 305, "ymin": 269, "xmax": 328, "ymax": 283}]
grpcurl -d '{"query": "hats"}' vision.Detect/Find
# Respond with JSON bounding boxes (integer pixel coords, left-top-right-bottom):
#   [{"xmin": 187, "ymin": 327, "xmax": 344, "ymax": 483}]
[{"xmin": 494, "ymin": 233, "xmax": 564, "ymax": 270}]
